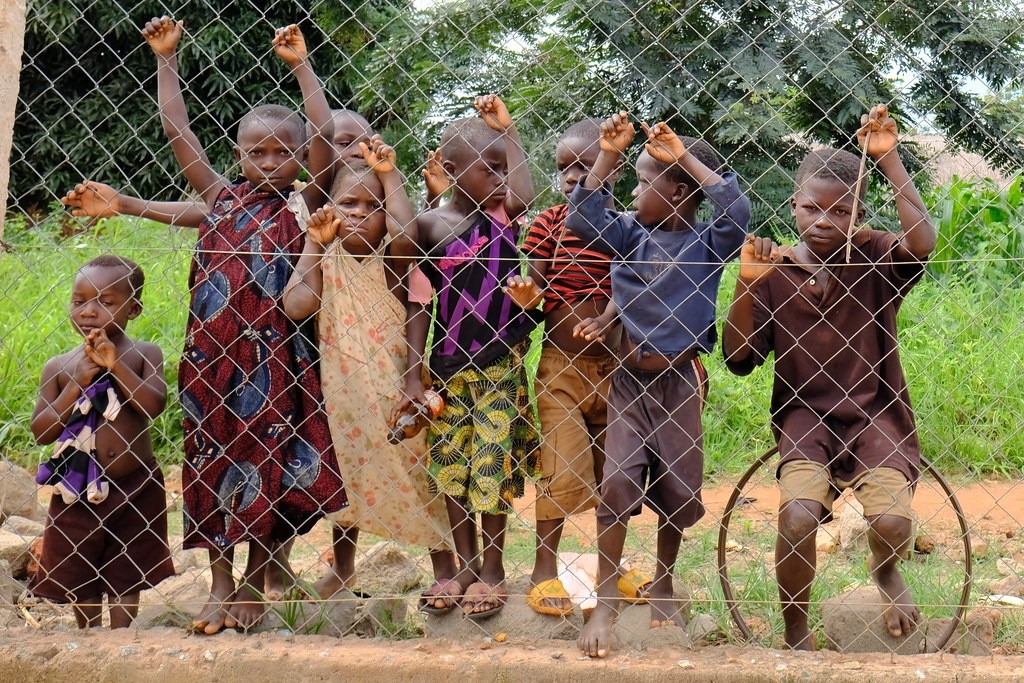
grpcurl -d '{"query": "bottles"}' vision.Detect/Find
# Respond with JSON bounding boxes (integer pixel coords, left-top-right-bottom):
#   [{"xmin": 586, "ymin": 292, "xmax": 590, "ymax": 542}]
[{"xmin": 387, "ymin": 383, "xmax": 448, "ymax": 445}]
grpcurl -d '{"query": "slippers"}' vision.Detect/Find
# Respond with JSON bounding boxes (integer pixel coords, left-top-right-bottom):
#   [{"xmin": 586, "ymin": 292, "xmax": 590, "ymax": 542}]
[
  {"xmin": 417, "ymin": 578, "xmax": 465, "ymax": 613},
  {"xmin": 525, "ymin": 578, "xmax": 573, "ymax": 615},
  {"xmin": 461, "ymin": 583, "xmax": 509, "ymax": 618},
  {"xmin": 594, "ymin": 568, "xmax": 653, "ymax": 603}
]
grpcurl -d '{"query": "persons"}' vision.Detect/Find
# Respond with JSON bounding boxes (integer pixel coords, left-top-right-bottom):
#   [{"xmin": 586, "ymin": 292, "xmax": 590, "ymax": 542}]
[
  {"xmin": 28, "ymin": 254, "xmax": 177, "ymax": 631},
  {"xmin": 721, "ymin": 103, "xmax": 936, "ymax": 652},
  {"xmin": 60, "ymin": 15, "xmax": 751, "ymax": 660}
]
